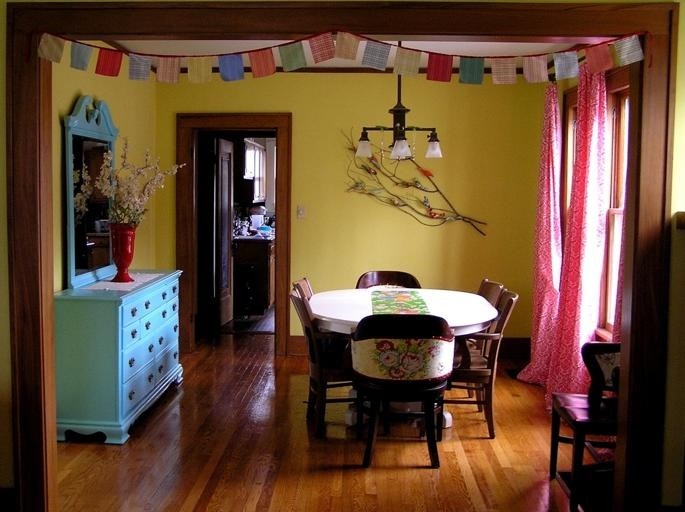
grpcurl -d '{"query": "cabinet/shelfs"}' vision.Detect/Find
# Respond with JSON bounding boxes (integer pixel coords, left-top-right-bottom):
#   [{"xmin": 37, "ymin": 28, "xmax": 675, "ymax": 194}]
[{"xmin": 56, "ymin": 267, "xmax": 182, "ymax": 444}]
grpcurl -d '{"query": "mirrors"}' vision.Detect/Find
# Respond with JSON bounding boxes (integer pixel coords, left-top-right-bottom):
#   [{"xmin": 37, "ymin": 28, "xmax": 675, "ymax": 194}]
[{"xmin": 63, "ymin": 93, "xmax": 121, "ymax": 289}]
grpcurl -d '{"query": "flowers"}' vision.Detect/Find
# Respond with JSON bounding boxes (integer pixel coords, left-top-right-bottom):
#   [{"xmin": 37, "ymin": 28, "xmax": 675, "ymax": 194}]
[
  {"xmin": 94, "ymin": 134, "xmax": 187, "ymax": 221},
  {"xmin": 74, "ymin": 162, "xmax": 94, "ymax": 226}
]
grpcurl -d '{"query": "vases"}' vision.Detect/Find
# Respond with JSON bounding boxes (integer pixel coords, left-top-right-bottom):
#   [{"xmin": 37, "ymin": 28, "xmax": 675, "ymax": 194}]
[{"xmin": 108, "ymin": 220, "xmax": 139, "ymax": 284}]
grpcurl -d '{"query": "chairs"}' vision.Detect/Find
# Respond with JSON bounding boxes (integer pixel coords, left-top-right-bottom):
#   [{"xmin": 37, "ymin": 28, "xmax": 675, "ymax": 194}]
[
  {"xmin": 293, "ymin": 276, "xmax": 315, "ymax": 301},
  {"xmin": 548, "ymin": 341, "xmax": 619, "ymax": 511},
  {"xmin": 356, "ymin": 270, "xmax": 422, "ymax": 290},
  {"xmin": 452, "ymin": 286, "xmax": 519, "ymax": 441},
  {"xmin": 348, "ymin": 315, "xmax": 453, "ymax": 472},
  {"xmin": 288, "ymin": 285, "xmax": 352, "ymax": 446},
  {"xmin": 452, "ymin": 278, "xmax": 504, "ymax": 399}
]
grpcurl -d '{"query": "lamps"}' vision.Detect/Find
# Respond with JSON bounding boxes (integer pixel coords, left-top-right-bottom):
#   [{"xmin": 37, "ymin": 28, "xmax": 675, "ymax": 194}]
[{"xmin": 356, "ymin": 42, "xmax": 444, "ymax": 160}]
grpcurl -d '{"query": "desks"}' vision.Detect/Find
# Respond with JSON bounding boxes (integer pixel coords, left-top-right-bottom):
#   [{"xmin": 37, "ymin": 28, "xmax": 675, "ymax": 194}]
[{"xmin": 309, "ymin": 284, "xmax": 498, "ymax": 437}]
